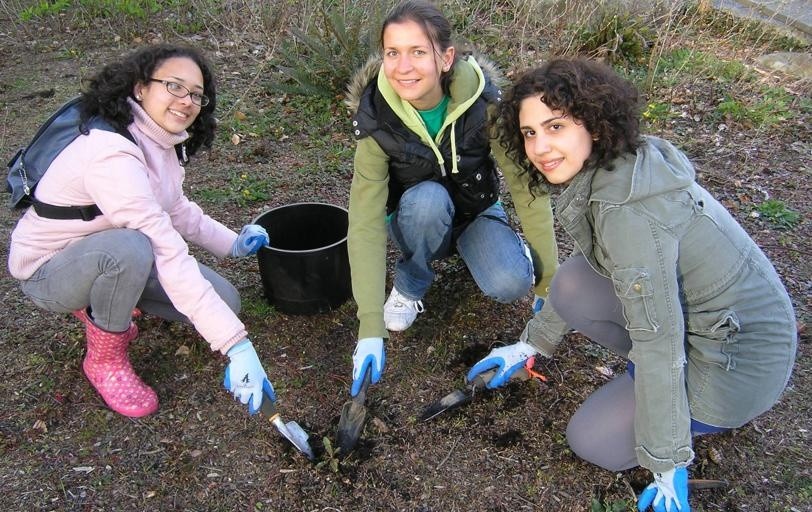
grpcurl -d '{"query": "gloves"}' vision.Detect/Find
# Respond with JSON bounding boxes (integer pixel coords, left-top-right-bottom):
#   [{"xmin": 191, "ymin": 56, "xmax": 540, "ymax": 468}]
[
  {"xmin": 638, "ymin": 466, "xmax": 690, "ymax": 512},
  {"xmin": 229, "ymin": 225, "xmax": 270, "ymax": 257},
  {"xmin": 224, "ymin": 339, "xmax": 275, "ymax": 415},
  {"xmin": 351, "ymin": 337, "xmax": 384, "ymax": 397},
  {"xmin": 531, "ymin": 293, "xmax": 548, "ymax": 315},
  {"xmin": 467, "ymin": 341, "xmax": 539, "ymax": 389}
]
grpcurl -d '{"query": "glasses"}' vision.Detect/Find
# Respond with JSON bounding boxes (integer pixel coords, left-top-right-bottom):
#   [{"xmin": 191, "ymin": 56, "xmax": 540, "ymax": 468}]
[{"xmin": 149, "ymin": 78, "xmax": 210, "ymax": 107}]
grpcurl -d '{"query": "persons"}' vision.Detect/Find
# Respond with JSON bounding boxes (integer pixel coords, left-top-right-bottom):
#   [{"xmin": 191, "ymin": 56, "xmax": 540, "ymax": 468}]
[
  {"xmin": 345, "ymin": 3, "xmax": 562, "ymax": 398},
  {"xmin": 7, "ymin": 43, "xmax": 277, "ymax": 419},
  {"xmin": 466, "ymin": 58, "xmax": 798, "ymax": 512}
]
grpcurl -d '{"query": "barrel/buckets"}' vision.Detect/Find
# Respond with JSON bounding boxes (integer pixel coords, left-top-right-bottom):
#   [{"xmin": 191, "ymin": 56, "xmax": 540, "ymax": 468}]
[{"xmin": 251, "ymin": 203, "xmax": 350, "ymax": 314}]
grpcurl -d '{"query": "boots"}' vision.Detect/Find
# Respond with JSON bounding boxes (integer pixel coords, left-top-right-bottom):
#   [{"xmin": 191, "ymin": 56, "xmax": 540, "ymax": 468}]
[{"xmin": 72, "ymin": 306, "xmax": 159, "ymax": 417}]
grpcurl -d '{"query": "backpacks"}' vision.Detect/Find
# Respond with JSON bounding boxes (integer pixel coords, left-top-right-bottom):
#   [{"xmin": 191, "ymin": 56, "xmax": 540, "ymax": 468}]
[{"xmin": 6, "ymin": 97, "xmax": 138, "ymax": 220}]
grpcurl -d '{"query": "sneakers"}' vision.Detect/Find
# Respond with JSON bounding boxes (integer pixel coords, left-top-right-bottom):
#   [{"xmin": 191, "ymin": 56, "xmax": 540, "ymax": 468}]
[{"xmin": 383, "ymin": 286, "xmax": 419, "ymax": 332}]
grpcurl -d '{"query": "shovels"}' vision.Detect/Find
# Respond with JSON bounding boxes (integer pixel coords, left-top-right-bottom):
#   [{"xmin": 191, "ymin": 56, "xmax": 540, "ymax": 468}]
[
  {"xmin": 334, "ymin": 364, "xmax": 373, "ymax": 458},
  {"xmin": 260, "ymin": 391, "xmax": 318, "ymax": 462},
  {"xmin": 421, "ymin": 367, "xmax": 531, "ymax": 422}
]
